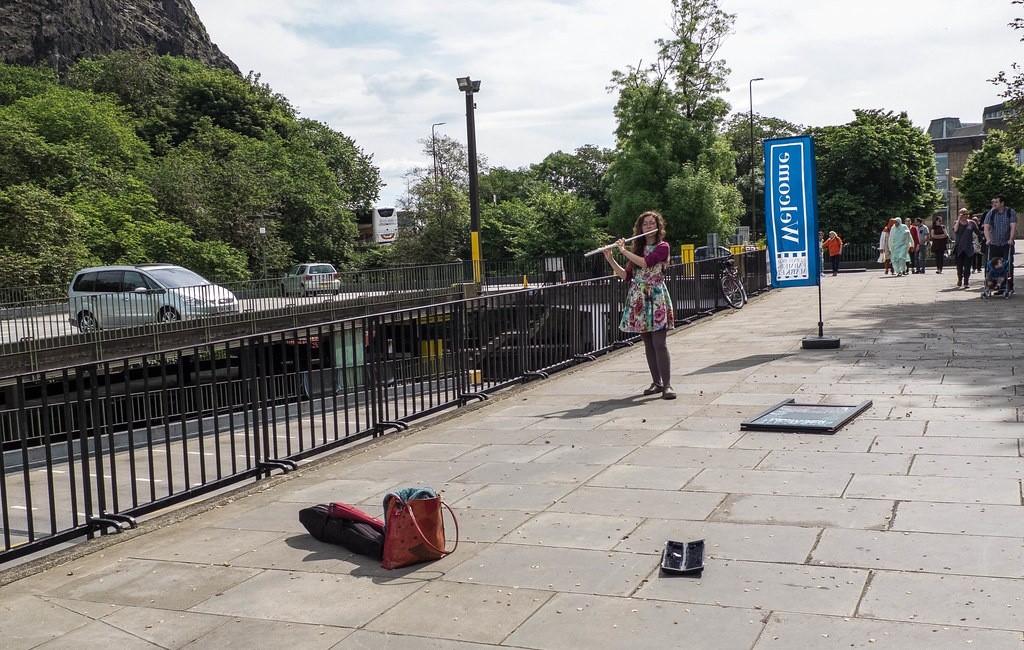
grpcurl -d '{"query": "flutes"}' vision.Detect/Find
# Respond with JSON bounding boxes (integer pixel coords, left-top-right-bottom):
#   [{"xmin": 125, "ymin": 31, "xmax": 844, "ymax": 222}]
[{"xmin": 583, "ymin": 228, "xmax": 660, "ymax": 258}]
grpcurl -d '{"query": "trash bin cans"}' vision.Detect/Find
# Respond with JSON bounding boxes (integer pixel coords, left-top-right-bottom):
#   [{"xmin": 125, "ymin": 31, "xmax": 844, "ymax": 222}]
[{"xmin": 732, "ymin": 250, "xmax": 770, "ymax": 297}]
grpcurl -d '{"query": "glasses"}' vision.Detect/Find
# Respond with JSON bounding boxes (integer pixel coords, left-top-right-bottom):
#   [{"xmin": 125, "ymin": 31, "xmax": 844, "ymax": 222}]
[
  {"xmin": 937, "ymin": 220, "xmax": 941, "ymax": 222},
  {"xmin": 963, "ymin": 213, "xmax": 968, "ymax": 216}
]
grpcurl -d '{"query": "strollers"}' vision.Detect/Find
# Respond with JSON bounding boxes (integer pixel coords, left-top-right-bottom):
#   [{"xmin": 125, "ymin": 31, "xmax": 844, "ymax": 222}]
[{"xmin": 980, "ymin": 240, "xmax": 1014, "ymax": 299}]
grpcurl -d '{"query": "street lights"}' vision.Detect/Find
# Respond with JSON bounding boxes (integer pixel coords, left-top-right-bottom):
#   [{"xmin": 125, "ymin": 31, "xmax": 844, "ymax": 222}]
[
  {"xmin": 749, "ymin": 77, "xmax": 764, "ymax": 243},
  {"xmin": 948, "ymin": 190, "xmax": 959, "ymax": 219},
  {"xmin": 944, "ymin": 168, "xmax": 951, "ymax": 236},
  {"xmin": 457, "ymin": 75, "xmax": 487, "ymax": 284},
  {"xmin": 432, "ymin": 122, "xmax": 447, "ymax": 196}
]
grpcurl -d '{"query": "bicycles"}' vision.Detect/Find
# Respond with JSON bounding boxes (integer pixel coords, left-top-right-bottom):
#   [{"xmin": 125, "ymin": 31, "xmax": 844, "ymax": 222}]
[{"xmin": 717, "ymin": 256, "xmax": 750, "ymax": 309}]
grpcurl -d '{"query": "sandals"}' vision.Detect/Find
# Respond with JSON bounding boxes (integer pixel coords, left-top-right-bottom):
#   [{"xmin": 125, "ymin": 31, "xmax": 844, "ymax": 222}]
[
  {"xmin": 662, "ymin": 386, "xmax": 676, "ymax": 399},
  {"xmin": 644, "ymin": 382, "xmax": 663, "ymax": 395}
]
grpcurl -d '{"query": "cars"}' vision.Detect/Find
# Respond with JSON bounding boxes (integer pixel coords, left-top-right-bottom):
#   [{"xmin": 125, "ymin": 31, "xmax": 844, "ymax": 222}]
[
  {"xmin": 67, "ymin": 263, "xmax": 240, "ymax": 334},
  {"xmin": 663, "ymin": 246, "xmax": 735, "ymax": 276},
  {"xmin": 280, "ymin": 263, "xmax": 341, "ymax": 299}
]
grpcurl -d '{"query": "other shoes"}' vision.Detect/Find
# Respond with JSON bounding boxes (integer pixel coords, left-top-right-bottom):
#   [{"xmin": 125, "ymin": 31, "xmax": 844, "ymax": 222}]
[
  {"xmin": 885, "ymin": 267, "xmax": 925, "ymax": 277},
  {"xmin": 957, "ymin": 279, "xmax": 962, "ymax": 286},
  {"xmin": 935, "ymin": 269, "xmax": 943, "ymax": 274},
  {"xmin": 832, "ymin": 271, "xmax": 839, "ymax": 276},
  {"xmin": 964, "ymin": 282, "xmax": 970, "ymax": 288},
  {"xmin": 978, "ymin": 270, "xmax": 980, "ymax": 273},
  {"xmin": 972, "ymin": 268, "xmax": 976, "ymax": 273}
]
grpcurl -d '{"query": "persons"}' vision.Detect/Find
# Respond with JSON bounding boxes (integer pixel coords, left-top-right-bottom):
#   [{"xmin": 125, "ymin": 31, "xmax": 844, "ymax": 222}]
[
  {"xmin": 877, "ymin": 217, "xmax": 930, "ymax": 278},
  {"xmin": 930, "ymin": 216, "xmax": 949, "ymax": 274},
  {"xmin": 819, "ymin": 231, "xmax": 843, "ymax": 276},
  {"xmin": 952, "ymin": 195, "xmax": 1017, "ymax": 295},
  {"xmin": 602, "ymin": 212, "xmax": 677, "ymax": 400}
]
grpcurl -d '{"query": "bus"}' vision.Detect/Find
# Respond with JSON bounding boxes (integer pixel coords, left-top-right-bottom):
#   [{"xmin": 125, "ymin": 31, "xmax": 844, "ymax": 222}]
[{"xmin": 349, "ymin": 206, "xmax": 397, "ymax": 250}]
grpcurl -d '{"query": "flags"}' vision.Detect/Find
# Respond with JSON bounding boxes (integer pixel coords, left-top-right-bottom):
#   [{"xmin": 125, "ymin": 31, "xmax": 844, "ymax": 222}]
[{"xmin": 764, "ymin": 136, "xmax": 818, "ymax": 288}]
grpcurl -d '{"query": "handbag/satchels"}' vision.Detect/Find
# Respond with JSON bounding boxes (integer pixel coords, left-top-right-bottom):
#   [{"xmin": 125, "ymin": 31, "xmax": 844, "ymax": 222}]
[
  {"xmin": 380, "ymin": 487, "xmax": 459, "ymax": 570},
  {"xmin": 328, "ymin": 499, "xmax": 385, "ymax": 530}
]
